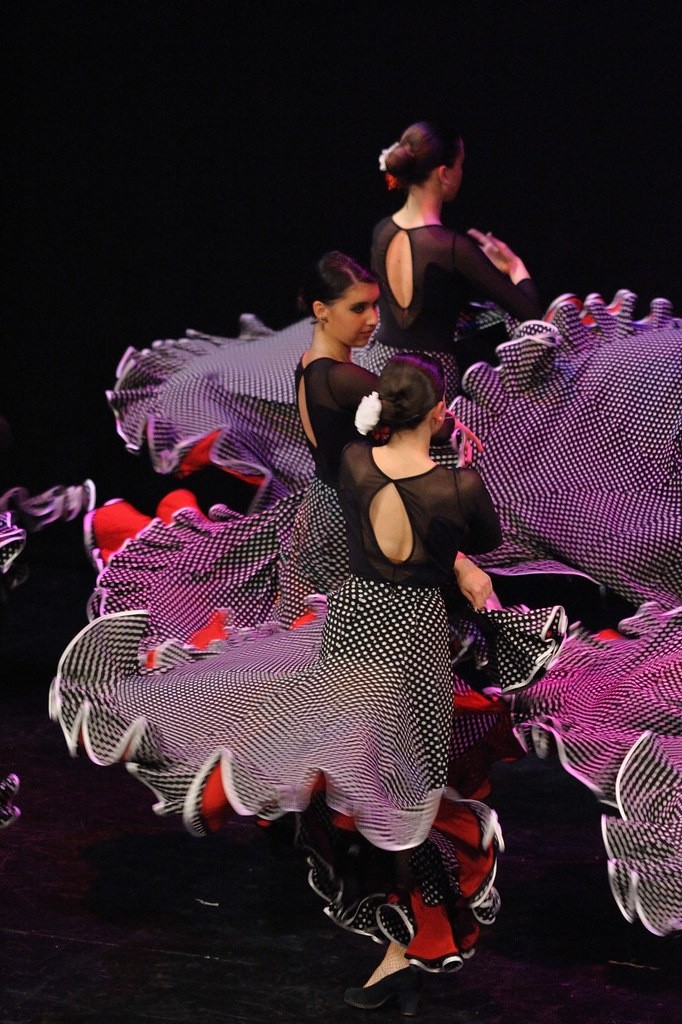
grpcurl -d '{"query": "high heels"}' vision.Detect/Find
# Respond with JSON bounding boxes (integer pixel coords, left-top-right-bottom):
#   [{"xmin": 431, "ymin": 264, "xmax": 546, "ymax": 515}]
[{"xmin": 344, "ymin": 966, "xmax": 423, "ymax": 1015}]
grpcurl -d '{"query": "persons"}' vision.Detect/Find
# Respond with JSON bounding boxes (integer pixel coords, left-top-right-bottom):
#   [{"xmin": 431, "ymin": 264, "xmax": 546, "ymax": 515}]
[{"xmin": 0, "ymin": 119, "xmax": 682, "ymax": 1010}]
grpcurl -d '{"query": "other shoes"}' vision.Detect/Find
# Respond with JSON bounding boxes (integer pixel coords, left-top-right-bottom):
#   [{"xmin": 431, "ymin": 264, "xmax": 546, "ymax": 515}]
[{"xmin": 0, "ymin": 775, "xmax": 20, "ymax": 830}]
